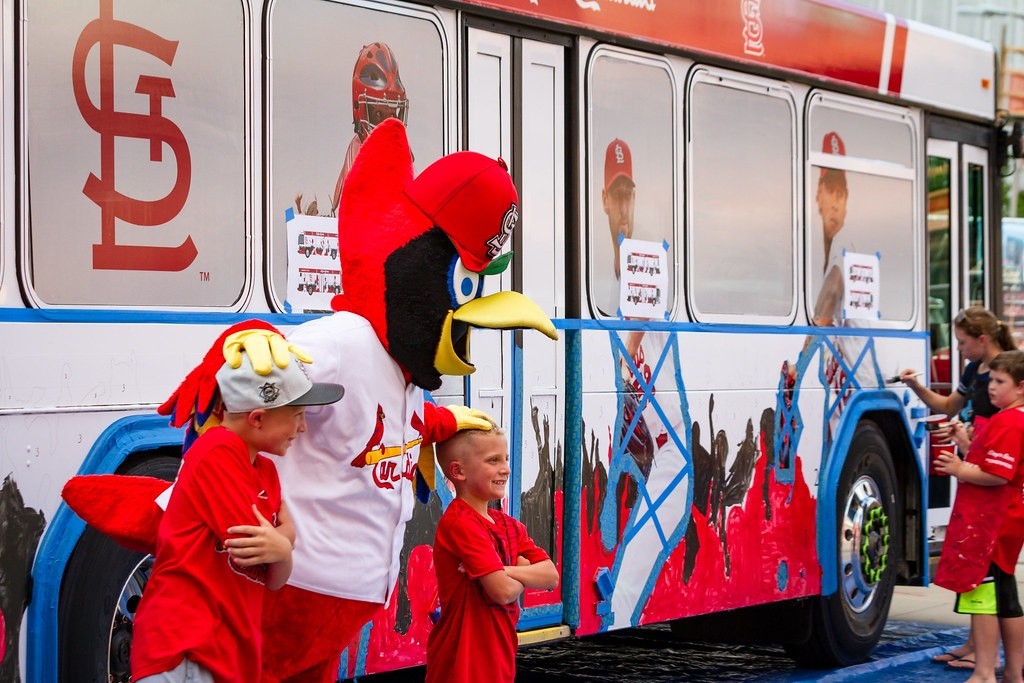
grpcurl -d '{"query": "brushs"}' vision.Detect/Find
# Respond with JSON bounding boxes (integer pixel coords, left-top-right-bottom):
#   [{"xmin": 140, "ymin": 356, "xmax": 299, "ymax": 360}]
[
  {"xmin": 924, "ymin": 421, "xmax": 957, "ymax": 431},
  {"xmin": 885, "ymin": 371, "xmax": 924, "ymax": 384}
]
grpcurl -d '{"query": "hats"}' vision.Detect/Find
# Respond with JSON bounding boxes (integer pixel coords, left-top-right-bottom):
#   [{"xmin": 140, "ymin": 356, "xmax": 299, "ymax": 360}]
[
  {"xmin": 820, "ymin": 132, "xmax": 845, "ymax": 179},
  {"xmin": 404, "ymin": 151, "xmax": 518, "ymax": 274},
  {"xmin": 216, "ymin": 348, "xmax": 344, "ymax": 413},
  {"xmin": 603, "ymin": 137, "xmax": 635, "ymax": 190}
]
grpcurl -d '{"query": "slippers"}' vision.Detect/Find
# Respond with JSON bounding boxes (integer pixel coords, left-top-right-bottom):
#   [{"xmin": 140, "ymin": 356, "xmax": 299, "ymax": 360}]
[
  {"xmin": 946, "ymin": 658, "xmax": 1001, "ymax": 673},
  {"xmin": 930, "ymin": 651, "xmax": 963, "ymax": 665}
]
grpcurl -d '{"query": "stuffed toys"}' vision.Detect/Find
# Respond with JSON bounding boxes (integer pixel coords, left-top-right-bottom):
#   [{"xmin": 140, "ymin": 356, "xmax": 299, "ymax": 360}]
[{"xmin": 62, "ymin": 118, "xmax": 561, "ymax": 683}]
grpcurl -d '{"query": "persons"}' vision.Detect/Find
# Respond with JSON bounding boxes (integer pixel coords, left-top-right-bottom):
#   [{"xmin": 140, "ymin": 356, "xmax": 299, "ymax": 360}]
[
  {"xmin": 331, "ymin": 42, "xmax": 414, "ymax": 206},
  {"xmin": 898, "ymin": 307, "xmax": 1024, "ymax": 683},
  {"xmin": 602, "ymin": 138, "xmax": 694, "ymax": 630},
  {"xmin": 783, "ymin": 132, "xmax": 887, "ymax": 446},
  {"xmin": 131, "ymin": 349, "xmax": 345, "ymax": 683},
  {"xmin": 425, "ymin": 420, "xmax": 561, "ymax": 683}
]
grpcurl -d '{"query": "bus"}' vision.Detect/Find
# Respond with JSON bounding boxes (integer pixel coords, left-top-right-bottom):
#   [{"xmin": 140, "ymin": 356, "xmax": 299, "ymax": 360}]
[{"xmin": 0, "ymin": 0, "xmax": 1024, "ymax": 683}]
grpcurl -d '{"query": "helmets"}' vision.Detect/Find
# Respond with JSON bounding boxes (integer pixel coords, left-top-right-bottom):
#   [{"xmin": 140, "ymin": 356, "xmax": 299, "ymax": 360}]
[{"xmin": 353, "ymin": 41, "xmax": 406, "ymax": 143}]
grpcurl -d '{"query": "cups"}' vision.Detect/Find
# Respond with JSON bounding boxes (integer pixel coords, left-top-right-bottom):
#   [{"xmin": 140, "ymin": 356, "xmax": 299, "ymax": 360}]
[
  {"xmin": 932, "ymin": 441, "xmax": 956, "ymax": 475},
  {"xmin": 926, "ymin": 414, "xmax": 952, "ymax": 445}
]
